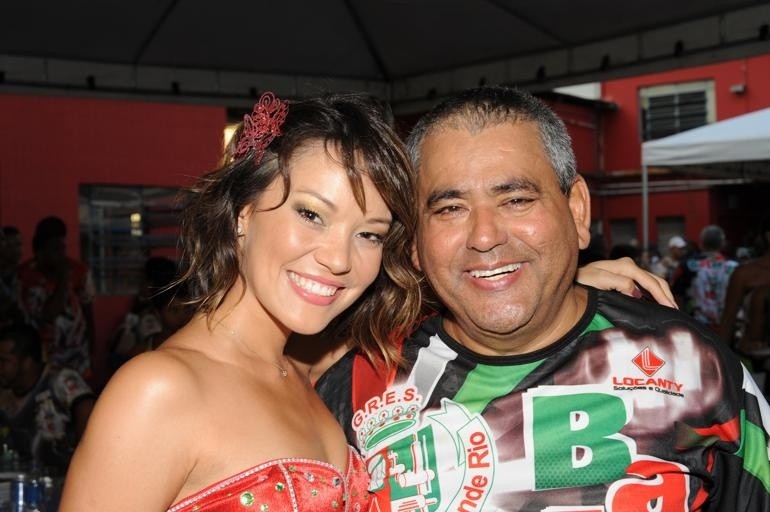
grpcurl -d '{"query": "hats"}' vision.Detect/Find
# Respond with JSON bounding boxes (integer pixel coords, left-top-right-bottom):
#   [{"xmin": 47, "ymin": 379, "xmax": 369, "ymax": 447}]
[{"xmin": 668, "ymin": 235, "xmax": 688, "ymax": 250}]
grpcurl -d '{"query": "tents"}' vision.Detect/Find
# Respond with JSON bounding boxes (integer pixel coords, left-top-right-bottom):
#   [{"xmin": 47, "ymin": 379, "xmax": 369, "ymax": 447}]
[{"xmin": 641, "ymin": 108, "xmax": 769, "ymax": 266}]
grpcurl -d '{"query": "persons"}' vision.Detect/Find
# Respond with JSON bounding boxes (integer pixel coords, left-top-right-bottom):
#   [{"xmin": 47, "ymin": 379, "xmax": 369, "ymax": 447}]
[
  {"xmin": 314, "ymin": 85, "xmax": 770, "ymax": 512},
  {"xmin": 1, "ymin": 216, "xmax": 193, "ymax": 489},
  {"xmin": 58, "ymin": 94, "xmax": 678, "ymax": 512},
  {"xmin": 586, "ymin": 224, "xmax": 770, "ymax": 404}
]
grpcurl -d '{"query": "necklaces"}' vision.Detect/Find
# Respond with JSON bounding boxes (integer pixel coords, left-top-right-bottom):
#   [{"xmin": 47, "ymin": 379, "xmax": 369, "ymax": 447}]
[{"xmin": 211, "ymin": 317, "xmax": 289, "ymax": 376}]
